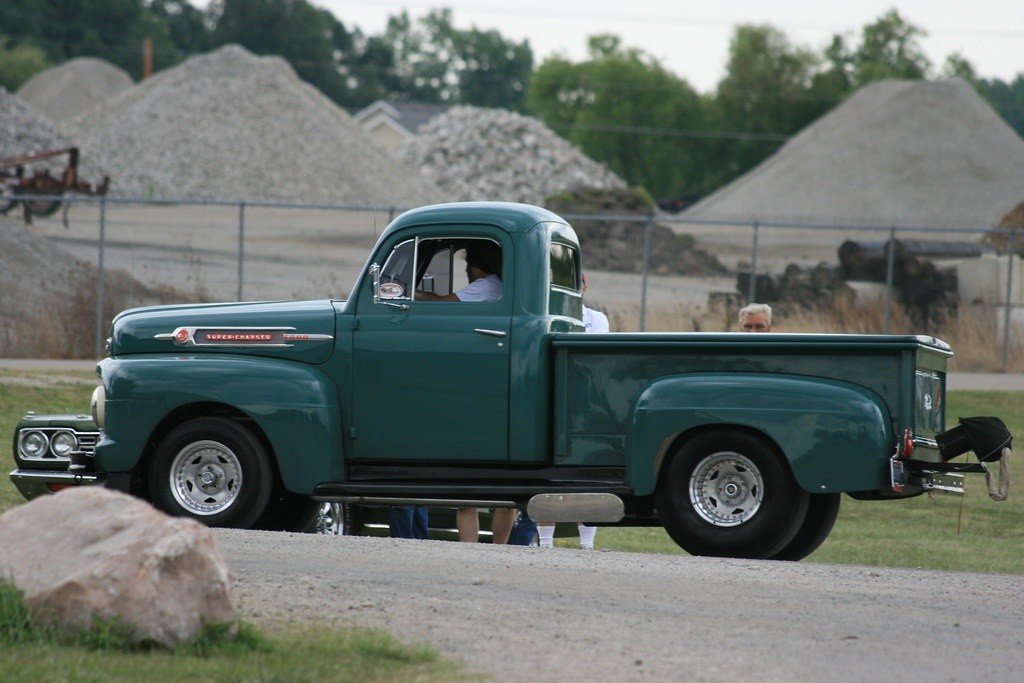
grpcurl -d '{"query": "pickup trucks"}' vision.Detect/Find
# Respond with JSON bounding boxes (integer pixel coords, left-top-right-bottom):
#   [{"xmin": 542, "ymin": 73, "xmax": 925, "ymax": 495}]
[
  {"xmin": 9, "ymin": 411, "xmax": 531, "ymax": 539},
  {"xmin": 90, "ymin": 201, "xmax": 1012, "ymax": 563}
]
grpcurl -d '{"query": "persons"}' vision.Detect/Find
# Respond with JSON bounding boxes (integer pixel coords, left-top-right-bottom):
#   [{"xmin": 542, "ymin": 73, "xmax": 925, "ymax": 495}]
[
  {"xmin": 737, "ymin": 303, "xmax": 773, "ymax": 332},
  {"xmin": 384, "ymin": 272, "xmax": 610, "ymax": 550},
  {"xmin": 390, "ymin": 243, "xmax": 502, "ymax": 302}
]
